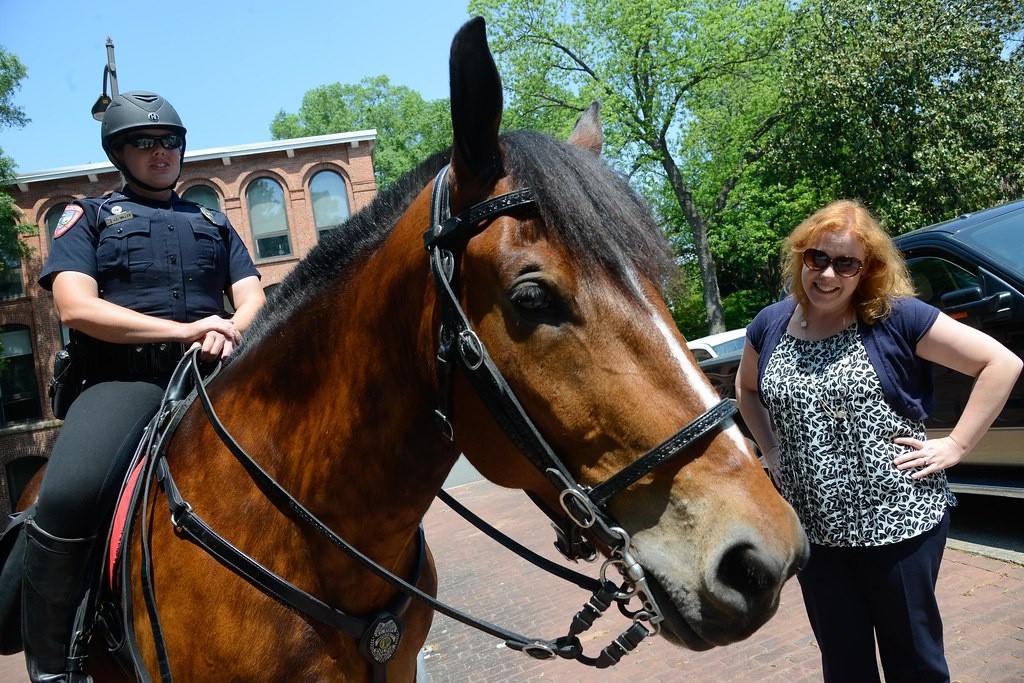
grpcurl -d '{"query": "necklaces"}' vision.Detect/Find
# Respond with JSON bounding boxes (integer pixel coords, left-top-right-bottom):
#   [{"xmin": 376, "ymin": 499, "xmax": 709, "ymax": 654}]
[{"xmin": 799, "ymin": 310, "xmax": 854, "ymax": 419}]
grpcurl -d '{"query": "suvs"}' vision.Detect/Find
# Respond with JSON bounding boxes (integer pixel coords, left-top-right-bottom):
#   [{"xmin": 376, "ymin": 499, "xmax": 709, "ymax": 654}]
[{"xmin": 881, "ymin": 198, "xmax": 1024, "ymax": 507}]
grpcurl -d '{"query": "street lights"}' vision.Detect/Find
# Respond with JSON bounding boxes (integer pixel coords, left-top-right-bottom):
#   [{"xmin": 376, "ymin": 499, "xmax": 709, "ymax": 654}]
[{"xmin": 89, "ymin": 36, "xmax": 130, "ymax": 192}]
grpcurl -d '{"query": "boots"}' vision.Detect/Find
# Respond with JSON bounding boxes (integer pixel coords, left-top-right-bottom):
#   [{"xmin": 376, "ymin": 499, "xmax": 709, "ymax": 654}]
[{"xmin": 20, "ymin": 514, "xmax": 98, "ymax": 683}]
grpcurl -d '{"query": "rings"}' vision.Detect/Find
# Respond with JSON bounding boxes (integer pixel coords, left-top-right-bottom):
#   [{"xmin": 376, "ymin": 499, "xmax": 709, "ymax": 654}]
[{"xmin": 923, "ymin": 458, "xmax": 926, "ymax": 466}]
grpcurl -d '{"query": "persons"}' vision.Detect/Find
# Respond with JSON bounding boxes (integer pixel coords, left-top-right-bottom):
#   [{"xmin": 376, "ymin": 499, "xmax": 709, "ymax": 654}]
[
  {"xmin": 19, "ymin": 91, "xmax": 266, "ymax": 683},
  {"xmin": 733, "ymin": 199, "xmax": 1024, "ymax": 683}
]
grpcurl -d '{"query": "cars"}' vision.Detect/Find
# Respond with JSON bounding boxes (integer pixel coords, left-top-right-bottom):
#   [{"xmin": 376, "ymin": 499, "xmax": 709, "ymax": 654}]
[
  {"xmin": 691, "ymin": 349, "xmax": 763, "ymax": 459},
  {"xmin": 681, "ymin": 326, "xmax": 750, "ymax": 365}
]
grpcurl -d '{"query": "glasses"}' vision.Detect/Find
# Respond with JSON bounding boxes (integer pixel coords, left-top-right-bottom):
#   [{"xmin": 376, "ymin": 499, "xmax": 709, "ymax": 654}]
[
  {"xmin": 803, "ymin": 248, "xmax": 863, "ymax": 279},
  {"xmin": 109, "ymin": 134, "xmax": 182, "ymax": 151}
]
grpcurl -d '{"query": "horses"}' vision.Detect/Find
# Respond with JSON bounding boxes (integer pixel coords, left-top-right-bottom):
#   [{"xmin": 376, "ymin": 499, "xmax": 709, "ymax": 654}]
[{"xmin": 17, "ymin": 18, "xmax": 809, "ymax": 682}]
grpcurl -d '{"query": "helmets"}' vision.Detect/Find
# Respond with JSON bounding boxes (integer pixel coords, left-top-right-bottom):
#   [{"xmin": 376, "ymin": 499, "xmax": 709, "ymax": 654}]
[{"xmin": 100, "ymin": 90, "xmax": 187, "ymax": 138}]
